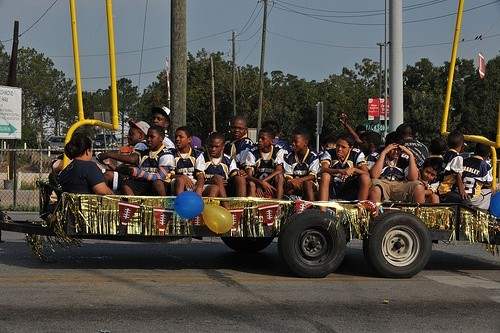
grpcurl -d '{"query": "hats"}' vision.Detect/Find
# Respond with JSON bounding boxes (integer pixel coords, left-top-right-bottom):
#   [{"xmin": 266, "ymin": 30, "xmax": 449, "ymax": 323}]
[
  {"xmin": 128, "ymin": 120, "xmax": 150, "ymax": 135},
  {"xmin": 151, "ymin": 105, "xmax": 172, "ymax": 127}
]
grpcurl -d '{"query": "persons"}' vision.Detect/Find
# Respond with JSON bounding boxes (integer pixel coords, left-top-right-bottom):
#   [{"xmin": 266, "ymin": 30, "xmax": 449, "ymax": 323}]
[
  {"xmin": 283, "ymin": 130, "xmax": 321, "ymax": 202},
  {"xmin": 167, "ymin": 126, "xmax": 204, "ymax": 197},
  {"xmin": 417, "ymin": 158, "xmax": 443, "ymax": 204},
  {"xmin": 248, "ymin": 129, "xmax": 283, "ymax": 198},
  {"xmin": 149, "ymin": 106, "xmax": 175, "ymax": 148},
  {"xmin": 396, "ymin": 123, "xmax": 428, "ymax": 169},
  {"xmin": 223, "ymin": 116, "xmax": 255, "ymax": 170},
  {"xmin": 463, "ymin": 142, "xmax": 493, "ymax": 211},
  {"xmin": 196, "ymin": 131, "xmax": 247, "ymax": 198},
  {"xmin": 427, "ymin": 137, "xmax": 446, "ymax": 158},
  {"xmin": 365, "ymin": 131, "xmax": 425, "ymax": 203},
  {"xmin": 438, "ymin": 130, "xmax": 473, "ymax": 207},
  {"xmin": 321, "ymin": 131, "xmax": 369, "ymax": 201},
  {"xmin": 47, "ymin": 133, "xmax": 115, "ymax": 195},
  {"xmin": 119, "ymin": 124, "xmax": 175, "ymax": 196},
  {"xmin": 259, "ymin": 119, "xmax": 289, "ymax": 151},
  {"xmin": 354, "ymin": 124, "xmax": 367, "ymax": 141},
  {"xmin": 95, "ymin": 120, "xmax": 150, "ymax": 179},
  {"xmin": 337, "ymin": 112, "xmax": 381, "ymax": 160}
]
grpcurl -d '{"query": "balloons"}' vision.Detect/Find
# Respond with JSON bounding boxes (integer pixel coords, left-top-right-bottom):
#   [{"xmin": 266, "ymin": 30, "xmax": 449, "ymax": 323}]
[
  {"xmin": 203, "ymin": 204, "xmax": 233, "ymax": 234},
  {"xmin": 489, "ymin": 191, "xmax": 500, "ymax": 217},
  {"xmin": 189, "ymin": 135, "xmax": 202, "ymax": 149},
  {"xmin": 174, "ymin": 191, "xmax": 205, "ymax": 218}
]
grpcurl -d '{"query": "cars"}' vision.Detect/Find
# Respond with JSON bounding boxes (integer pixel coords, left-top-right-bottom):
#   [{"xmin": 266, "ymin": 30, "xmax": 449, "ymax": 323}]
[
  {"xmin": 46, "ymin": 135, "xmax": 67, "ymax": 153},
  {"xmin": 92, "ymin": 134, "xmax": 120, "ymax": 155}
]
